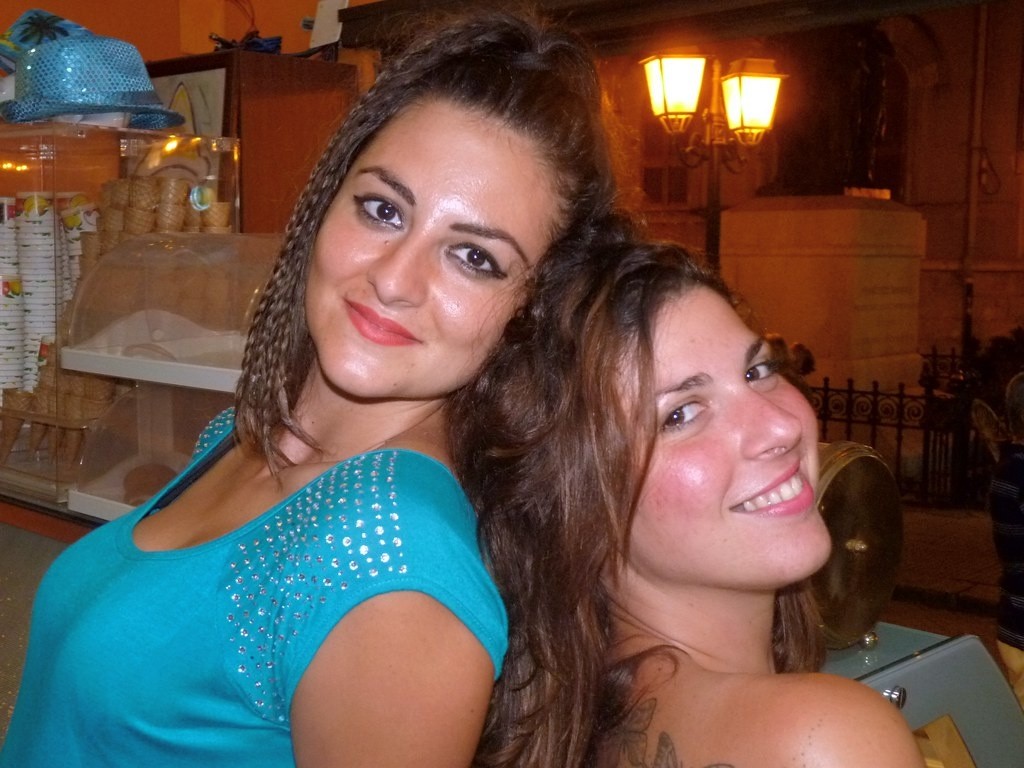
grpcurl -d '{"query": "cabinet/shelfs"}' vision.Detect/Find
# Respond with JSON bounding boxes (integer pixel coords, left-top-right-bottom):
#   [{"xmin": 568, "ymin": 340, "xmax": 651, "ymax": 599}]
[{"xmin": 144, "ymin": 52, "xmax": 361, "ymax": 236}]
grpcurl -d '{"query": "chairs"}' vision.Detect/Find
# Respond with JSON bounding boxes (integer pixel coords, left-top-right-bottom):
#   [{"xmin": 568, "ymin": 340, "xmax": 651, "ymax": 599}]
[{"xmin": 972, "ymin": 398, "xmax": 1011, "ymax": 518}]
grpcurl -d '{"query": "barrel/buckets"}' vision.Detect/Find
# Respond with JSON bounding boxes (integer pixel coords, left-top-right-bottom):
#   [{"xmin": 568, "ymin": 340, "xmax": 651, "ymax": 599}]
[{"xmin": 798, "ymin": 443, "xmax": 903, "ymax": 648}]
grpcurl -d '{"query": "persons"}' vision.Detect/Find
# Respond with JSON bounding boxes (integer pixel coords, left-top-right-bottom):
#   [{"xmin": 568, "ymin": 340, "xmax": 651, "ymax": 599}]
[
  {"xmin": 447, "ymin": 216, "xmax": 931, "ymax": 768},
  {"xmin": 0, "ymin": 15, "xmax": 612, "ymax": 768}
]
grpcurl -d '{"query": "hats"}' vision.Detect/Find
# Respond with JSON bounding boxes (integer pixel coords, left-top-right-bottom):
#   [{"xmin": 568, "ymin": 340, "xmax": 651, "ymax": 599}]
[{"xmin": 0, "ymin": 38, "xmax": 187, "ymax": 130}]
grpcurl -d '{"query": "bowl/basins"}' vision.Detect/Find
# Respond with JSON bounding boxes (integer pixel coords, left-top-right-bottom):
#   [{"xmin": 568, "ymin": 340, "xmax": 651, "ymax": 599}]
[{"xmin": 0, "ymin": 191, "xmax": 101, "ymax": 408}]
